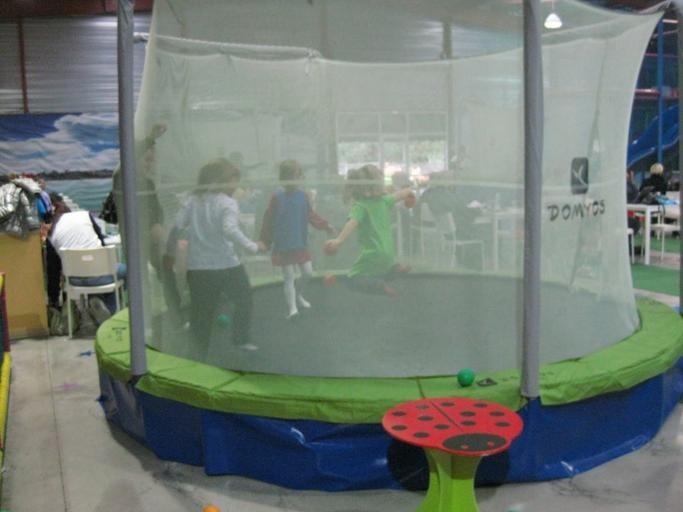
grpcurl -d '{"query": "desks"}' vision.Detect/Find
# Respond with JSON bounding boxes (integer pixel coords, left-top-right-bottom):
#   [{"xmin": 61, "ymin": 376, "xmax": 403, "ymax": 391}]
[
  {"xmin": 626, "ymin": 203, "xmax": 666, "ymax": 264},
  {"xmin": 105, "ymin": 234, "xmax": 123, "ymax": 265},
  {"xmin": 381, "ymin": 397, "xmax": 524, "ymax": 512}
]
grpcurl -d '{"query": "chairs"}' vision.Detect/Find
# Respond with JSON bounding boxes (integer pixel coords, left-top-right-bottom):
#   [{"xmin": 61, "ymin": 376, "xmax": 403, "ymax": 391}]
[
  {"xmin": 397, "ymin": 193, "xmax": 524, "ymax": 270},
  {"xmin": 238, "ymin": 190, "xmax": 317, "ymax": 277},
  {"xmin": 58, "ymin": 246, "xmax": 125, "ymax": 338}
]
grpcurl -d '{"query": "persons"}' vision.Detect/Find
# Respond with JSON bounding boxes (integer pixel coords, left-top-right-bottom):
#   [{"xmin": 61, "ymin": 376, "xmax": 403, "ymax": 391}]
[
  {"xmin": 113, "ymin": 123, "xmax": 182, "ymax": 342},
  {"xmin": 626, "ymin": 169, "xmax": 640, "ymax": 256},
  {"xmin": 42, "ymin": 192, "xmax": 127, "ymax": 327},
  {"xmin": 639, "ymin": 163, "xmax": 667, "ymax": 236}
]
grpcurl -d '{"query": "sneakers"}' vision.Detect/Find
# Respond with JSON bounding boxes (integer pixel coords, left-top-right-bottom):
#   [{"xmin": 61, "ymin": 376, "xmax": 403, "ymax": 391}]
[{"xmin": 89, "ymin": 296, "xmax": 111, "ymax": 320}]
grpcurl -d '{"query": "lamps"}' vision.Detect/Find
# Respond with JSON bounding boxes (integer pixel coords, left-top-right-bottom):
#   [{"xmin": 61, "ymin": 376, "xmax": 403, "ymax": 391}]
[{"xmin": 543, "ymin": 1, "xmax": 561, "ymax": 29}]
